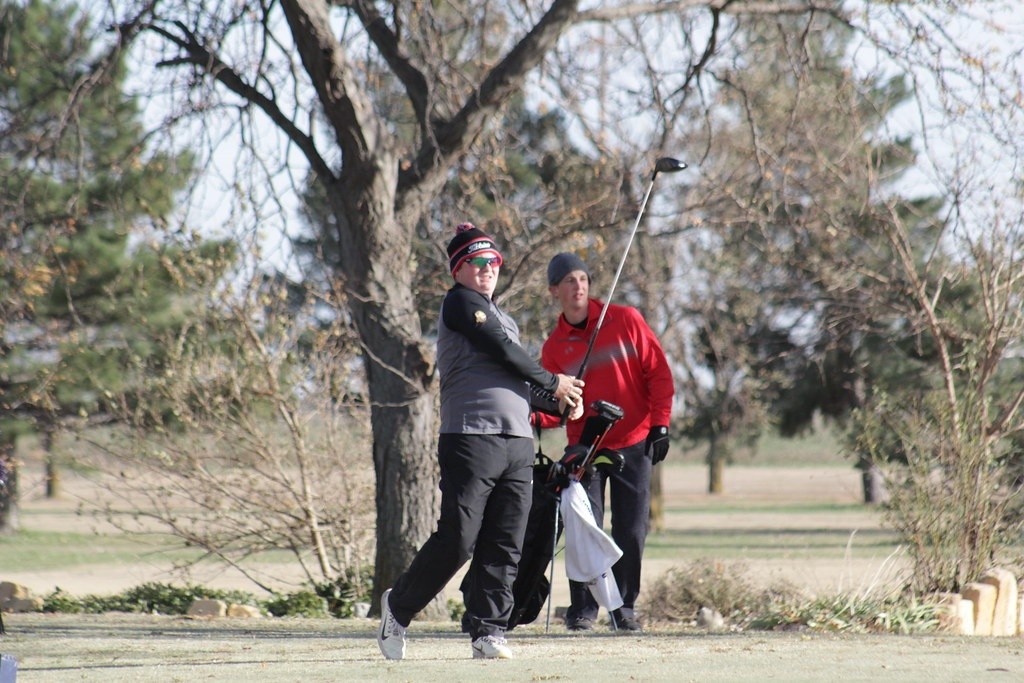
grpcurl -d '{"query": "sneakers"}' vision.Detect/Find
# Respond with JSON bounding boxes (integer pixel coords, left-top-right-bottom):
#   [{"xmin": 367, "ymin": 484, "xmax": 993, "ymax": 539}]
[
  {"xmin": 565, "ymin": 616, "xmax": 593, "ymax": 630},
  {"xmin": 471, "ymin": 635, "xmax": 512, "ymax": 659},
  {"xmin": 376, "ymin": 587, "xmax": 405, "ymax": 661},
  {"xmin": 608, "ymin": 617, "xmax": 641, "ymax": 630}
]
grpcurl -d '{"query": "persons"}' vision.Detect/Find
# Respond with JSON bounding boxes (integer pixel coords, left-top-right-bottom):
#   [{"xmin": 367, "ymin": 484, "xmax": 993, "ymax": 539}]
[
  {"xmin": 377, "ymin": 223, "xmax": 587, "ymax": 662},
  {"xmin": 528, "ymin": 249, "xmax": 675, "ymax": 634}
]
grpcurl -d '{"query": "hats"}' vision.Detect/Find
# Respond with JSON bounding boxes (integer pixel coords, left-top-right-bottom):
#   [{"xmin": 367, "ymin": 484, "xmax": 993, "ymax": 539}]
[
  {"xmin": 446, "ymin": 221, "xmax": 501, "ymax": 278},
  {"xmin": 547, "ymin": 250, "xmax": 594, "ymax": 287}
]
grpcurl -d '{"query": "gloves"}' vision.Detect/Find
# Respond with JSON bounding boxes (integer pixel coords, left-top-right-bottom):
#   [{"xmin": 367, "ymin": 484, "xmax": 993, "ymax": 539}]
[{"xmin": 642, "ymin": 423, "xmax": 669, "ymax": 464}]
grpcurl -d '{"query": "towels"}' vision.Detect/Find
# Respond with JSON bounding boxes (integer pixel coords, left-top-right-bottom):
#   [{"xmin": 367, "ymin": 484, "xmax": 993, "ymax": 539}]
[{"xmin": 560, "ymin": 476, "xmax": 626, "ymax": 614}]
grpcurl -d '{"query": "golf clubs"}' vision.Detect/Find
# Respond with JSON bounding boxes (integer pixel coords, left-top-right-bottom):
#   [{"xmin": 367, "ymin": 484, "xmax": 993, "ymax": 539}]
[
  {"xmin": 546, "ymin": 399, "xmax": 624, "ymax": 495},
  {"xmin": 559, "ymin": 157, "xmax": 688, "ymax": 428}
]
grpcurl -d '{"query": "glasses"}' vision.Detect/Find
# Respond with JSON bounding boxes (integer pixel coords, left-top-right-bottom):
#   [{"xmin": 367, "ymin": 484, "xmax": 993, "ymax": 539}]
[{"xmin": 464, "ymin": 255, "xmax": 501, "ymax": 269}]
